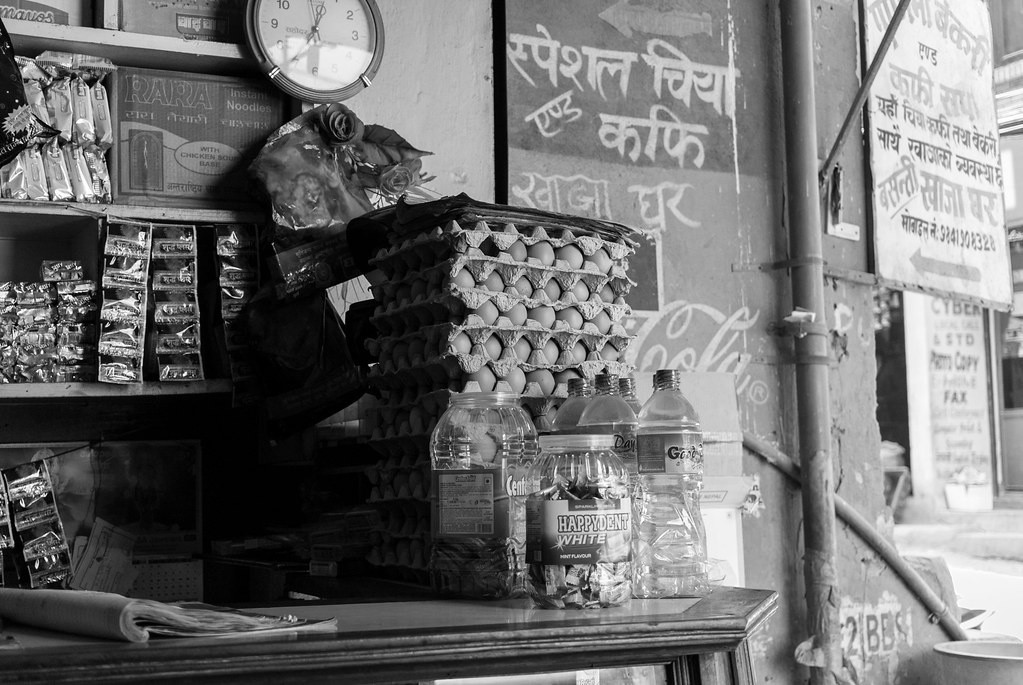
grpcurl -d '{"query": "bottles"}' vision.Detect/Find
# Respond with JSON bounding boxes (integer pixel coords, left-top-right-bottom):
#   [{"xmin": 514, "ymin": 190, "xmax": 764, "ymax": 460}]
[
  {"xmin": 633, "ymin": 369, "xmax": 711, "ymax": 599},
  {"xmin": 525, "ymin": 434, "xmax": 634, "ymax": 610},
  {"xmin": 429, "ymin": 391, "xmax": 538, "ymax": 601},
  {"xmin": 618, "ymin": 377, "xmax": 643, "ymax": 416},
  {"xmin": 575, "ymin": 373, "xmax": 639, "ymax": 601},
  {"xmin": 551, "ymin": 378, "xmax": 591, "ymax": 434}
]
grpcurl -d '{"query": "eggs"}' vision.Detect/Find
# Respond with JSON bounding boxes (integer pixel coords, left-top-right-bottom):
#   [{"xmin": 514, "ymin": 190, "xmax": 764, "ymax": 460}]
[
  {"xmin": 377, "ymin": 470, "xmax": 427, "ymax": 563},
  {"xmin": 374, "ymin": 239, "xmax": 618, "ymax": 435}
]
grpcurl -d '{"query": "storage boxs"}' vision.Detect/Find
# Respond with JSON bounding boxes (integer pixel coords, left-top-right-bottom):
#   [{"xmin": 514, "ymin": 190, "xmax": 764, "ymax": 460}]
[{"xmin": 632, "ymin": 371, "xmax": 745, "ymax": 479}]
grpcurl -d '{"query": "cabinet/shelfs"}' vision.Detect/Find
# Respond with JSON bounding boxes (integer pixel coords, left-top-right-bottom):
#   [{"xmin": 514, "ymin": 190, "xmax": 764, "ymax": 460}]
[{"xmin": 0, "ymin": 15, "xmax": 316, "ymax": 402}]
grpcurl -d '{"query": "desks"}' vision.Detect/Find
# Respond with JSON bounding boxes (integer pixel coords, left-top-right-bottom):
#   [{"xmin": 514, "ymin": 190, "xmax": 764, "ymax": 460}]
[{"xmin": 0, "ymin": 584, "xmax": 780, "ymax": 685}]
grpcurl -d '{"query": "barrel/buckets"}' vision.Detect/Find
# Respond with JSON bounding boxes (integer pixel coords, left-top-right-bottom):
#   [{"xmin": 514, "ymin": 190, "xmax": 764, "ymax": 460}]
[{"xmin": 933, "ymin": 639, "xmax": 1023, "ymax": 685}]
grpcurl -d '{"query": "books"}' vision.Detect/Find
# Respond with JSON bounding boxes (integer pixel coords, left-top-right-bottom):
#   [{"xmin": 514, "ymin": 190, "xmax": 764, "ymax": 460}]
[{"xmin": 0, "ymin": 586, "xmax": 338, "ymax": 650}]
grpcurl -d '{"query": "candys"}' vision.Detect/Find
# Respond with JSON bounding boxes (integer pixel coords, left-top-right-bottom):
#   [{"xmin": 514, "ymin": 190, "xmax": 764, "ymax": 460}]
[{"xmin": 431, "ymin": 472, "xmax": 633, "ymax": 608}]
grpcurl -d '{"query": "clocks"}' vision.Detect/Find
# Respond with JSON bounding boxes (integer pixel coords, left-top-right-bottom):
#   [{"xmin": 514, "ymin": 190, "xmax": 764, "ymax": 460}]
[{"xmin": 245, "ymin": 0, "xmax": 385, "ymax": 104}]
